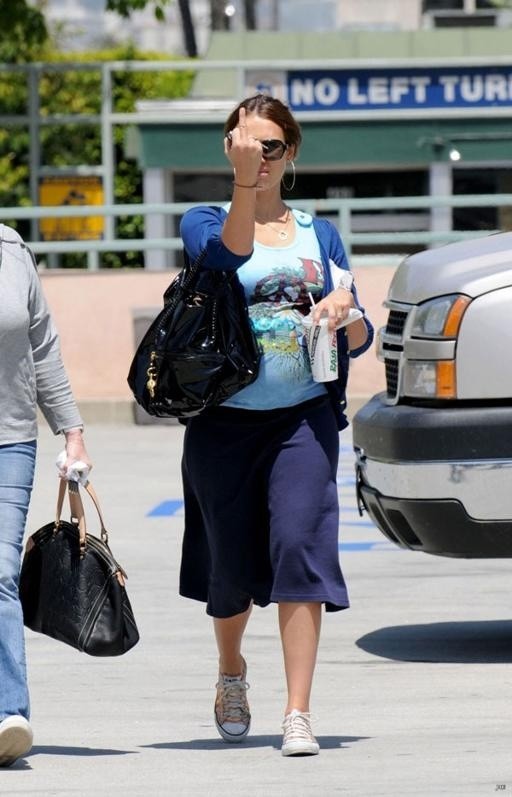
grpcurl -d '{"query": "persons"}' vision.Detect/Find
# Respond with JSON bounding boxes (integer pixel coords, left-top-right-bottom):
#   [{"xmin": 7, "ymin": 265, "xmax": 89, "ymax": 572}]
[
  {"xmin": 1, "ymin": 222, "xmax": 93, "ymax": 766},
  {"xmin": 182, "ymin": 95, "xmax": 374, "ymax": 759}
]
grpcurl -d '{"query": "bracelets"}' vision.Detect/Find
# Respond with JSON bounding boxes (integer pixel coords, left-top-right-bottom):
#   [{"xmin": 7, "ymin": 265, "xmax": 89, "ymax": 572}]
[{"xmin": 234, "ymin": 182, "xmax": 260, "ymax": 195}]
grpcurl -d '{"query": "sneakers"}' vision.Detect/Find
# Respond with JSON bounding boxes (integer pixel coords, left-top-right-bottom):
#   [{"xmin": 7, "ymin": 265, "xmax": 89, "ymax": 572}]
[
  {"xmin": 0, "ymin": 714, "xmax": 33, "ymax": 770},
  {"xmin": 282, "ymin": 709, "xmax": 320, "ymax": 756},
  {"xmin": 213, "ymin": 653, "xmax": 251, "ymax": 743}
]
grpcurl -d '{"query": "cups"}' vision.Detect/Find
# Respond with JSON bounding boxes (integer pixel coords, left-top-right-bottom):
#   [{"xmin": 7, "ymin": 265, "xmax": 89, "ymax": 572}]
[{"xmin": 303, "ymin": 314, "xmax": 342, "ymax": 383}]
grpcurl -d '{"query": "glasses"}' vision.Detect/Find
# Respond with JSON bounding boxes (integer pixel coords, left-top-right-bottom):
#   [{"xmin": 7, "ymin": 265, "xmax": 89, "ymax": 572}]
[{"xmin": 226, "ymin": 134, "xmax": 289, "ymax": 161}]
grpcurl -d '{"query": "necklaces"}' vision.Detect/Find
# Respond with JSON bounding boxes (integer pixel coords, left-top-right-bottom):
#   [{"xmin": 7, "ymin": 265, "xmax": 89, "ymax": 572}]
[{"xmin": 256, "ymin": 212, "xmax": 295, "ymax": 242}]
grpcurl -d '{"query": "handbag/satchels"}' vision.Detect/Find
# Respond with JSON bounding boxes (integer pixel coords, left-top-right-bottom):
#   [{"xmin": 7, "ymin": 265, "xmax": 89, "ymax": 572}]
[
  {"xmin": 128, "ymin": 238, "xmax": 260, "ymax": 418},
  {"xmin": 18, "ymin": 467, "xmax": 140, "ymax": 657}
]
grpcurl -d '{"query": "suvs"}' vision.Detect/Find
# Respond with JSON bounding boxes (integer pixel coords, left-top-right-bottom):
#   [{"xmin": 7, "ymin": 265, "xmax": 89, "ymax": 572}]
[{"xmin": 351, "ymin": 226, "xmax": 512, "ymax": 562}]
